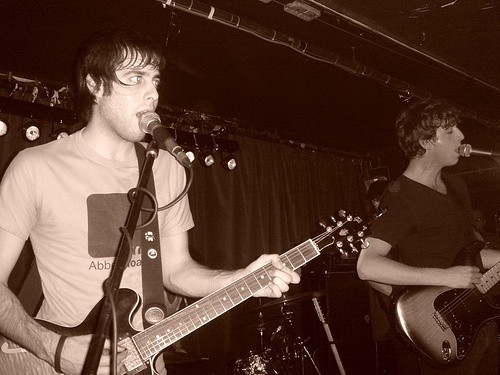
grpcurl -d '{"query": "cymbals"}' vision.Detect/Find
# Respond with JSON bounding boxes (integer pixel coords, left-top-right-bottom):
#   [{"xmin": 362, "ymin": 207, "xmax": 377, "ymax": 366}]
[
  {"xmin": 244, "ymin": 291, "xmax": 312, "ymax": 313},
  {"xmin": 163, "ymin": 351, "xmax": 201, "ymax": 364},
  {"xmin": 232, "ymin": 316, "xmax": 282, "ymax": 330}
]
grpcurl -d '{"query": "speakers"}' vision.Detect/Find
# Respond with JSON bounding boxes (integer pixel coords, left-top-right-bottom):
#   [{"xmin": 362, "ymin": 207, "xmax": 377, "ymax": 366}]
[{"xmin": 303, "ymin": 271, "xmax": 378, "ymax": 375}]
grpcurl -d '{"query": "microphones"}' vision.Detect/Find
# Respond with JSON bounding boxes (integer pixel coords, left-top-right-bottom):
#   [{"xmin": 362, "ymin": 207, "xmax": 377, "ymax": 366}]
[
  {"xmin": 460, "ymin": 143, "xmax": 500, "ymax": 158},
  {"xmin": 138, "ymin": 111, "xmax": 192, "ymax": 168}
]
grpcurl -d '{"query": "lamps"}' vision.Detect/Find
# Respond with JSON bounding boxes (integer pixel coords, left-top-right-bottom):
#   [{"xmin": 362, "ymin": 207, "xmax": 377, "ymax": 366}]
[
  {"xmin": 0, "ymin": 95, "xmax": 78, "ymax": 140},
  {"xmin": 162, "ymin": 126, "xmax": 240, "ymax": 171}
]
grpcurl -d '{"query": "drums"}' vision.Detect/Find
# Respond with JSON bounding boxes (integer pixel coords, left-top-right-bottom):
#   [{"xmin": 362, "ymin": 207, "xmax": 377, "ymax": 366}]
[{"xmin": 227, "ymin": 347, "xmax": 291, "ymax": 375}]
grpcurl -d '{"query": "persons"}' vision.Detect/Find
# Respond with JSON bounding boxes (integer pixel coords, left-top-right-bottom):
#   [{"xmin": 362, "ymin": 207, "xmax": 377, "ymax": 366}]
[
  {"xmin": 357, "ymin": 97, "xmax": 500, "ymax": 301},
  {"xmin": 1, "ymin": 28, "xmax": 301, "ymax": 375}
]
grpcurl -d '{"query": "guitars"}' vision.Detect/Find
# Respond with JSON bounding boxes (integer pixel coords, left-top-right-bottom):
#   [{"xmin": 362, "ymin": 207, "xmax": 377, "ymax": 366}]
[
  {"xmin": 392, "ymin": 261, "xmax": 500, "ymax": 368},
  {"xmin": 0, "ymin": 208, "xmax": 373, "ymax": 375}
]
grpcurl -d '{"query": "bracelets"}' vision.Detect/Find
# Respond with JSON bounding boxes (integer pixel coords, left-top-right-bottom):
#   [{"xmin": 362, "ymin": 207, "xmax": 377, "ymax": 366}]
[{"xmin": 53, "ymin": 335, "xmax": 67, "ymax": 373}]
